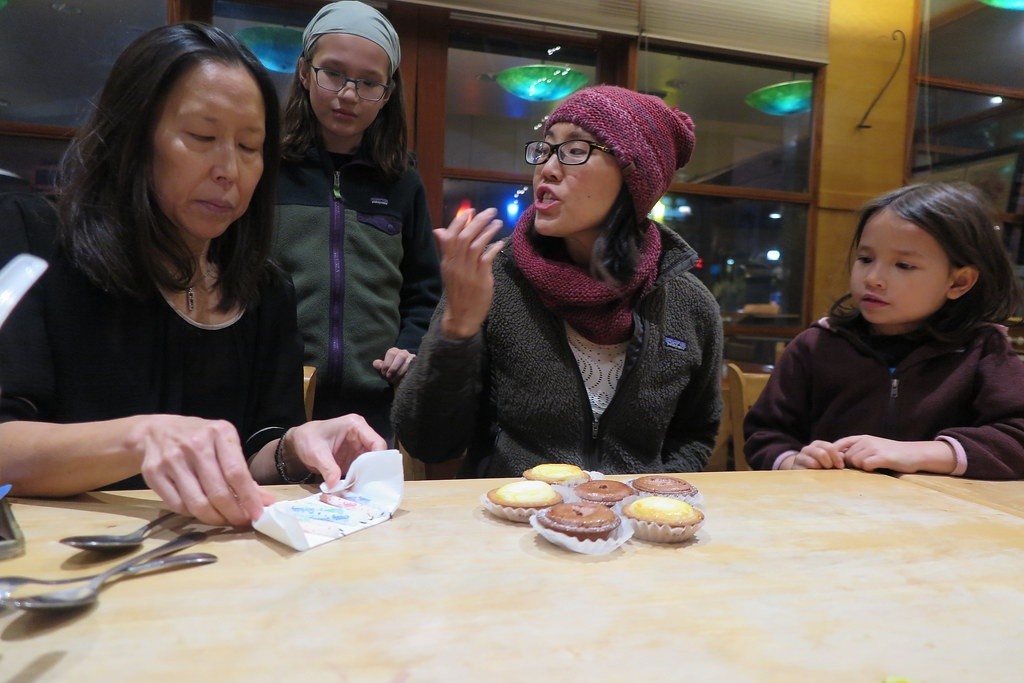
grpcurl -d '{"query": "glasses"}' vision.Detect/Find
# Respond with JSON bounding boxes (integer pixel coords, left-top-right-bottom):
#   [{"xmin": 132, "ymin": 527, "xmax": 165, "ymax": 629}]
[
  {"xmin": 524, "ymin": 140, "xmax": 615, "ymax": 165},
  {"xmin": 308, "ymin": 61, "xmax": 392, "ymax": 102}
]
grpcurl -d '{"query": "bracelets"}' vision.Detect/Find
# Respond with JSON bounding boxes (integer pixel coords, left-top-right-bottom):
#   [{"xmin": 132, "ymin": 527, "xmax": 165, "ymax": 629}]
[{"xmin": 275, "ymin": 431, "xmax": 315, "ymax": 484}]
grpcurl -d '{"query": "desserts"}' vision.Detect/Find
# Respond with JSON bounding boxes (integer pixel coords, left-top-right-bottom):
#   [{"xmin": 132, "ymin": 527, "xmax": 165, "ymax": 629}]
[{"xmin": 481, "ymin": 463, "xmax": 708, "ymax": 555}]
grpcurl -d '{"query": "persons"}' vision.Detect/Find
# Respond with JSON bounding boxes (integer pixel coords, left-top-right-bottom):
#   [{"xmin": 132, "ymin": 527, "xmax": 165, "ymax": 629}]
[
  {"xmin": 0, "ymin": 19, "xmax": 389, "ymax": 525},
  {"xmin": 269, "ymin": 0, "xmax": 441, "ymax": 447},
  {"xmin": 741, "ymin": 179, "xmax": 1024, "ymax": 479},
  {"xmin": 388, "ymin": 86, "xmax": 723, "ymax": 480}
]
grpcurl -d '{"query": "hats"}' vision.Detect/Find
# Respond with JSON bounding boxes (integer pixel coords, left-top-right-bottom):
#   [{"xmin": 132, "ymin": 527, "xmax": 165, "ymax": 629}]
[
  {"xmin": 546, "ymin": 86, "xmax": 695, "ymax": 223},
  {"xmin": 302, "ymin": 0, "xmax": 401, "ymax": 78}
]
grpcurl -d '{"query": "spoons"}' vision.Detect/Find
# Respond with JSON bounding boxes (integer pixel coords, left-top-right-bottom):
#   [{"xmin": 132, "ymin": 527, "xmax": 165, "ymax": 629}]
[
  {"xmin": 0, "ymin": 552, "xmax": 217, "ymax": 606},
  {"xmin": 60, "ymin": 508, "xmax": 210, "ymax": 557},
  {"xmin": 13, "ymin": 531, "xmax": 208, "ymax": 609}
]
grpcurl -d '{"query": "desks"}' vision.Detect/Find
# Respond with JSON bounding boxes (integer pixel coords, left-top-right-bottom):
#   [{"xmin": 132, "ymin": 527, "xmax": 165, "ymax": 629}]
[{"xmin": 0, "ymin": 468, "xmax": 1024, "ymax": 683}]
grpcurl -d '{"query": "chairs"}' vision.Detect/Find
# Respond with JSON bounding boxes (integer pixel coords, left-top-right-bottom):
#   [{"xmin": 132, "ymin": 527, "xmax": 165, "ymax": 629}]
[{"xmin": 729, "ymin": 362, "xmax": 761, "ymax": 470}]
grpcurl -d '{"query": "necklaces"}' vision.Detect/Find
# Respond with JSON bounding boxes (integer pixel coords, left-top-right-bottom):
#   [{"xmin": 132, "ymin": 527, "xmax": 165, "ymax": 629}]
[{"xmin": 185, "ymin": 262, "xmax": 215, "ymax": 311}]
